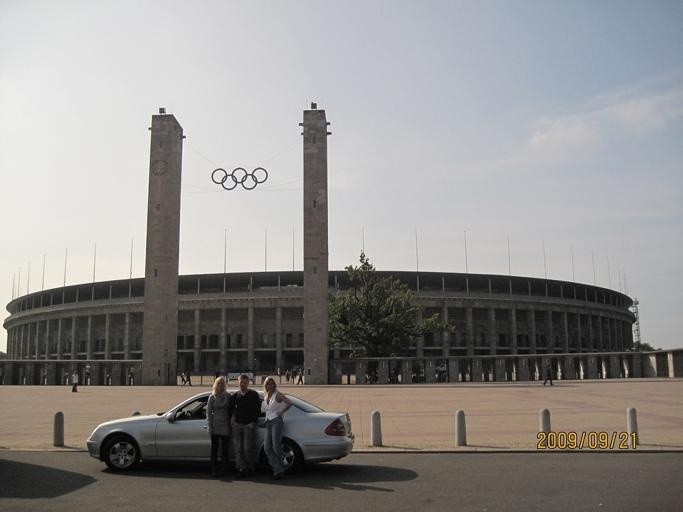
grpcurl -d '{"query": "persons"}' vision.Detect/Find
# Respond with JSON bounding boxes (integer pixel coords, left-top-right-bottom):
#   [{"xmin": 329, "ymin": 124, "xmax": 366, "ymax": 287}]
[
  {"xmin": 70, "ymin": 368, "xmax": 78, "ymax": 392},
  {"xmin": 183, "ymin": 369, "xmax": 192, "ymax": 386},
  {"xmin": 262, "ymin": 376, "xmax": 293, "ymax": 480},
  {"xmin": 180, "ymin": 368, "xmax": 186, "ymax": 385},
  {"xmin": 542, "ymin": 364, "xmax": 553, "ymax": 386},
  {"xmin": 214, "ymin": 365, "xmax": 426, "ymax": 386},
  {"xmin": 228, "ymin": 374, "xmax": 261, "ymax": 480},
  {"xmin": 205, "ymin": 374, "xmax": 232, "ymax": 477},
  {"xmin": 127, "ymin": 367, "xmax": 134, "ymax": 386}
]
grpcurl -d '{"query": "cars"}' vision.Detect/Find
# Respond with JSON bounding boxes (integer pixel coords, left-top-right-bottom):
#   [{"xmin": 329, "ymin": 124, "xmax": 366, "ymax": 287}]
[{"xmin": 85, "ymin": 391, "xmax": 354, "ymax": 473}]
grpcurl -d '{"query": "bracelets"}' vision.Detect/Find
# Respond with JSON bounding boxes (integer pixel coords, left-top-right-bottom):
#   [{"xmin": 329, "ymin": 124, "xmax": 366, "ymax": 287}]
[{"xmin": 279, "ymin": 408, "xmax": 283, "ymax": 414}]
